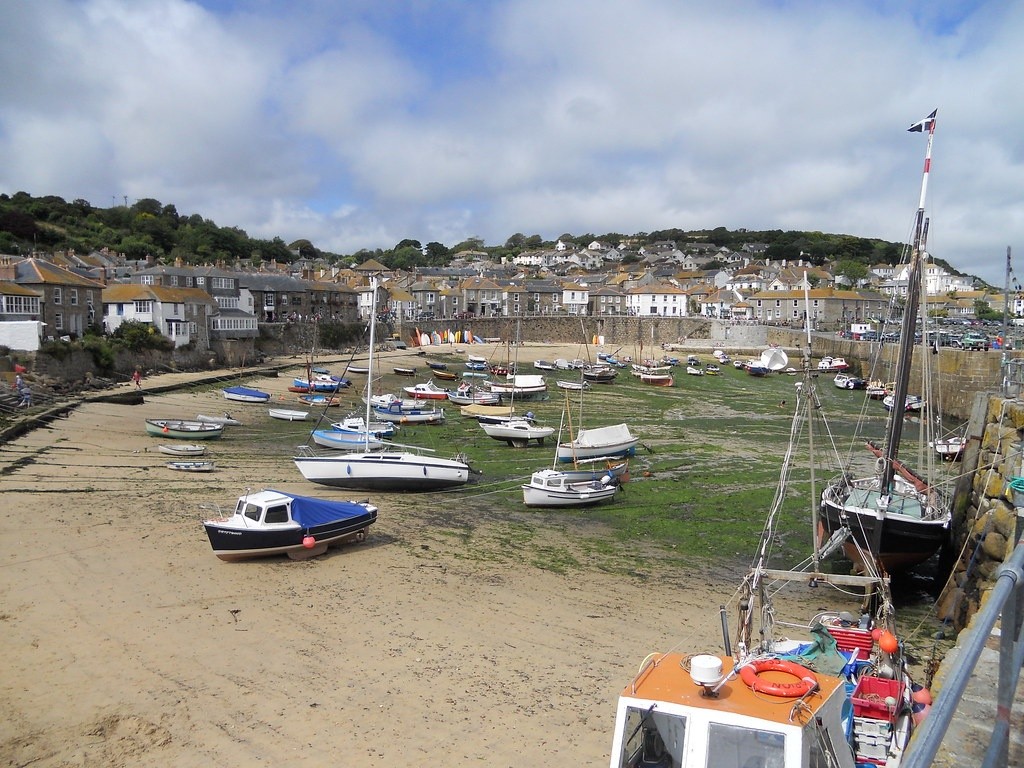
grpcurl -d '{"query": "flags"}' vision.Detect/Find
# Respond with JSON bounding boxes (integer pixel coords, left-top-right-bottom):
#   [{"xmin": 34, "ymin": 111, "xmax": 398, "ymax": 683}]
[{"xmin": 906, "ymin": 109, "xmax": 935, "ymax": 132}]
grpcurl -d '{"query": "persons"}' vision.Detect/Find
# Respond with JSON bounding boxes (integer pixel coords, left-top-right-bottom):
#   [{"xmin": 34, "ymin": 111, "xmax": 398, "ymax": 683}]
[
  {"xmin": 132, "ymin": 370, "xmax": 142, "ymax": 391},
  {"xmin": 15, "ymin": 372, "xmax": 31, "ymax": 408},
  {"xmin": 293, "ymin": 312, "xmax": 309, "ymax": 323},
  {"xmin": 309, "ymin": 312, "xmax": 322, "ymax": 324}
]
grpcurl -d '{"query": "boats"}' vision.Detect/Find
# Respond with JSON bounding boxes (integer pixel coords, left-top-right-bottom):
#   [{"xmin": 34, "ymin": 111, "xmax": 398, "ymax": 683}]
[
  {"xmin": 145, "ymin": 417, "xmax": 225, "ymax": 441},
  {"xmin": 296, "ymin": 394, "xmax": 342, "ymax": 407},
  {"xmin": 198, "ymin": 412, "xmax": 244, "ymax": 426},
  {"xmin": 223, "ymin": 383, "xmax": 273, "ymax": 404},
  {"xmin": 268, "ymin": 408, "xmax": 310, "ymax": 422},
  {"xmin": 167, "ymin": 462, "xmax": 216, "ymax": 473},
  {"xmin": 215, "ymin": 484, "xmax": 379, "ymax": 562},
  {"xmin": 289, "ymin": 274, "xmax": 484, "ymax": 494}
]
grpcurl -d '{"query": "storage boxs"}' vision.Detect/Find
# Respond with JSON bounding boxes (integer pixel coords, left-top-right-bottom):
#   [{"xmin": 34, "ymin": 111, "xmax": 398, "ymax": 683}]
[{"xmin": 827, "ymin": 628, "xmax": 906, "ymax": 766}]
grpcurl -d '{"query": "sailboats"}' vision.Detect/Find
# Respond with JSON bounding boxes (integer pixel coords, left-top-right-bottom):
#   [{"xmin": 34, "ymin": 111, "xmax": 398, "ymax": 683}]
[
  {"xmin": 609, "ymin": 269, "xmax": 932, "ymax": 767},
  {"xmin": 309, "ymin": 304, "xmax": 1023, "ymax": 602},
  {"xmin": 815, "ymin": 202, "xmax": 972, "ymax": 587}
]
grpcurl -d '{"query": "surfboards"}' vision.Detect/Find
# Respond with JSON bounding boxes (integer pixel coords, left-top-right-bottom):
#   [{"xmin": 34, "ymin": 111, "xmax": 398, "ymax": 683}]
[{"xmin": 415, "ymin": 326, "xmax": 482, "ymax": 345}]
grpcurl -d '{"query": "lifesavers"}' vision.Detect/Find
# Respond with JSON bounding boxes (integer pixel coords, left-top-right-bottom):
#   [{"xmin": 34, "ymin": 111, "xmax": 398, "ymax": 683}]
[
  {"xmin": 740, "ymin": 659, "xmax": 817, "ymax": 697},
  {"xmin": 491, "ymin": 369, "xmax": 507, "ymax": 374},
  {"xmin": 997, "ymin": 338, "xmax": 1003, "ymax": 346}
]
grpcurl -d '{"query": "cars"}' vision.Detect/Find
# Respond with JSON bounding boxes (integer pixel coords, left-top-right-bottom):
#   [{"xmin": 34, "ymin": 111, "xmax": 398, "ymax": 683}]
[
  {"xmin": 415, "ymin": 311, "xmax": 435, "ymax": 322},
  {"xmin": 454, "ymin": 311, "xmax": 475, "ymax": 320},
  {"xmin": 959, "ymin": 333, "xmax": 990, "ymax": 352}
]
